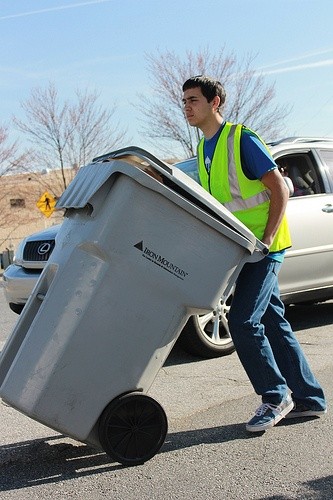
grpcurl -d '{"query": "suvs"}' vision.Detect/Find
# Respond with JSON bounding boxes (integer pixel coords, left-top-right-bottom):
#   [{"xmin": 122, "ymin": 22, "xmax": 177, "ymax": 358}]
[{"xmin": 2, "ymin": 137, "xmax": 333, "ymax": 357}]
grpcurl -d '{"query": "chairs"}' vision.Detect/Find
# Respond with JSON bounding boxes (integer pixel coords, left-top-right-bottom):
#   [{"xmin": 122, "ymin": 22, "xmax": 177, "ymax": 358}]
[{"xmin": 288, "ymin": 166, "xmax": 314, "ymax": 195}]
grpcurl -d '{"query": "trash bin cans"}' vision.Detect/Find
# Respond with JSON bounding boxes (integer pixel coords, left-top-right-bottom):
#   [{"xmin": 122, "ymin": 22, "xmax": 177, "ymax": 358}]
[
  {"xmin": 0, "ymin": 144, "xmax": 269, "ymax": 467},
  {"xmin": 0, "ymin": 253, "xmax": 4, "ymax": 268},
  {"xmin": 3, "ymin": 250, "xmax": 14, "ymax": 269}
]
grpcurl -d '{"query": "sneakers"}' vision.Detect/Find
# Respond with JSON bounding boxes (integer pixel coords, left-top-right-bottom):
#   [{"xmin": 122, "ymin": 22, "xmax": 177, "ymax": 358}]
[{"xmin": 246, "ymin": 394, "xmax": 328, "ymax": 432}]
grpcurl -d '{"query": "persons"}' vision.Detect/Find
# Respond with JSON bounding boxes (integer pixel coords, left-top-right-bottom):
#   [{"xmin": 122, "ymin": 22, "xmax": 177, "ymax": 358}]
[
  {"xmin": 275, "ymin": 159, "xmax": 304, "ymax": 197},
  {"xmin": 182, "ymin": 74, "xmax": 328, "ymax": 432}
]
